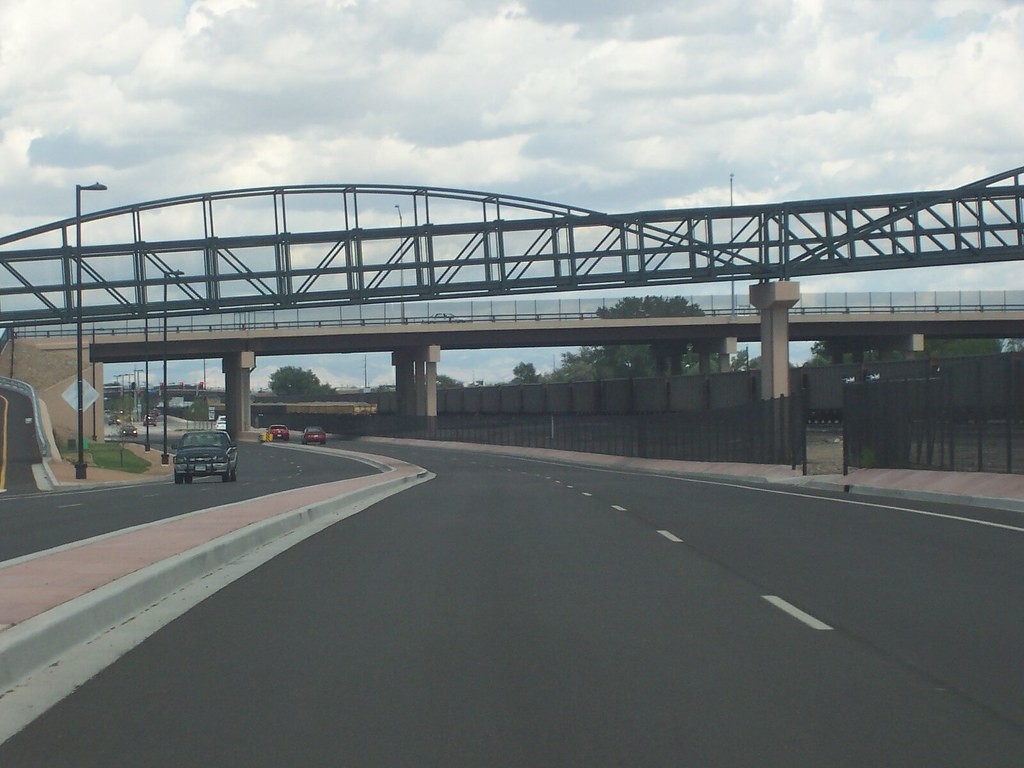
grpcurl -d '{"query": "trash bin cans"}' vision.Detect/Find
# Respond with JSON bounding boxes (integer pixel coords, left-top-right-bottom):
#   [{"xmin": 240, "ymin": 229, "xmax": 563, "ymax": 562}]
[
  {"xmin": 78, "ymin": 436, "xmax": 90, "ymax": 449},
  {"xmin": 68, "ymin": 439, "xmax": 77, "ymax": 450},
  {"xmin": 260, "ymin": 434, "xmax": 274, "ymax": 442}
]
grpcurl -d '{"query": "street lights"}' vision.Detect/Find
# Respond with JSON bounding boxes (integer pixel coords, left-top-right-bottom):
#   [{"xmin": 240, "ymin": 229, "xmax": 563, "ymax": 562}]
[
  {"xmin": 73, "ymin": 183, "xmax": 107, "ymax": 478},
  {"xmin": 113, "ymin": 370, "xmax": 144, "ymax": 421},
  {"xmin": 730, "ymin": 174, "xmax": 734, "ymax": 316},
  {"xmin": 395, "ymin": 205, "xmax": 404, "ymax": 325},
  {"xmin": 163, "ymin": 269, "xmax": 185, "ymax": 464}
]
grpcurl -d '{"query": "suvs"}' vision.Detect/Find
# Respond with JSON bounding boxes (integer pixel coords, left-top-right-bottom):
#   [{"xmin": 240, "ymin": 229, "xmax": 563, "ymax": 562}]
[
  {"xmin": 108, "ymin": 415, "xmax": 121, "ymax": 426},
  {"xmin": 143, "ymin": 414, "xmax": 157, "ymax": 426}
]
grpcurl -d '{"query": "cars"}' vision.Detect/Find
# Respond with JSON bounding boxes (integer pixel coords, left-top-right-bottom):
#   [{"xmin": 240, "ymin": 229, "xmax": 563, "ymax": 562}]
[
  {"xmin": 171, "ymin": 431, "xmax": 238, "ymax": 484},
  {"xmin": 266, "ymin": 425, "xmax": 289, "ymax": 441},
  {"xmin": 301, "ymin": 426, "xmax": 326, "ymax": 445},
  {"xmin": 214, "ymin": 415, "xmax": 226, "ymax": 431},
  {"xmin": 119, "ymin": 425, "xmax": 137, "ymax": 438}
]
